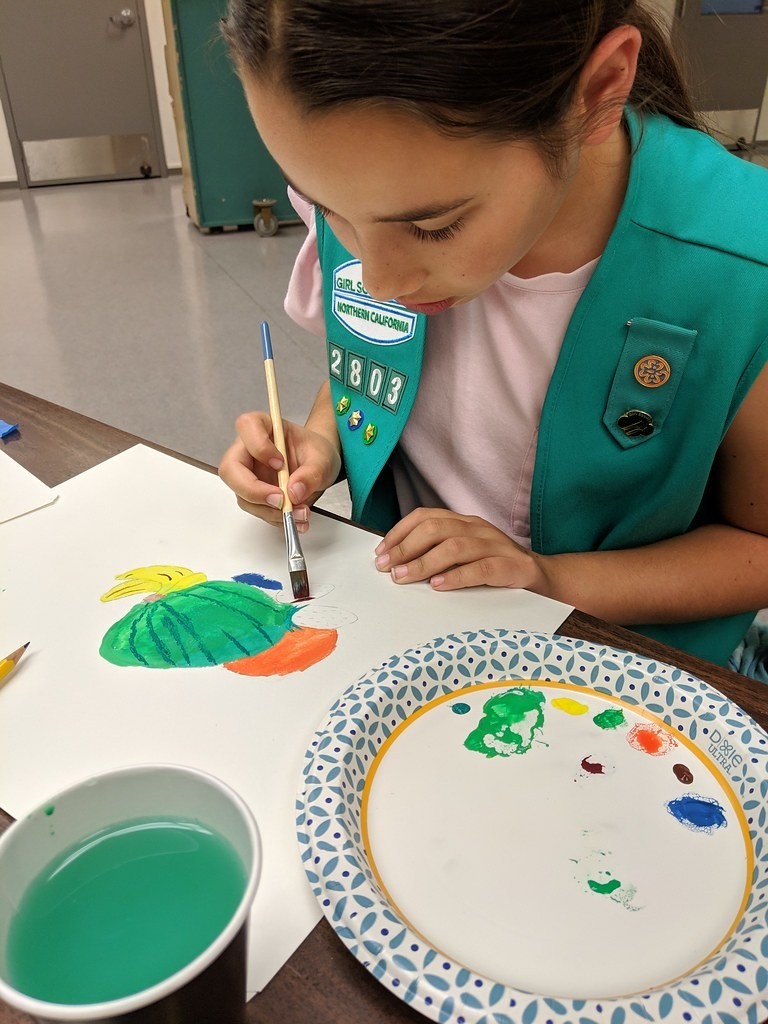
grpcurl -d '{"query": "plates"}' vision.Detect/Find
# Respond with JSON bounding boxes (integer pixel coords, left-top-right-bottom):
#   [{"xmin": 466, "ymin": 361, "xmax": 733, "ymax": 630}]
[{"xmin": 296, "ymin": 630, "xmax": 768, "ymax": 1024}]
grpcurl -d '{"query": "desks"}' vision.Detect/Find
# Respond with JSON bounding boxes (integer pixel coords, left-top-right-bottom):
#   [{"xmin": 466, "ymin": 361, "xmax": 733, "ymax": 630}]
[{"xmin": 0, "ymin": 378, "xmax": 768, "ymax": 1024}]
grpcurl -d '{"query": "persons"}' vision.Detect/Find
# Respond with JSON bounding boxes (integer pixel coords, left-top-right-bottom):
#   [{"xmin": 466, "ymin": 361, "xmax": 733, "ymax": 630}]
[{"xmin": 218, "ymin": 0, "xmax": 768, "ymax": 667}]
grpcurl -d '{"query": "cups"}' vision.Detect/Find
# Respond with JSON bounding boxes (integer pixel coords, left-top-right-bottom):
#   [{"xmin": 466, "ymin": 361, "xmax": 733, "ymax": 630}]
[{"xmin": 0, "ymin": 765, "xmax": 264, "ymax": 1024}]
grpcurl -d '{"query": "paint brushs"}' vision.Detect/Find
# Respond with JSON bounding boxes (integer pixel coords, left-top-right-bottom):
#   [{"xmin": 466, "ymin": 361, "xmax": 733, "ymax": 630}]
[{"xmin": 261, "ymin": 321, "xmax": 309, "ymax": 600}]
